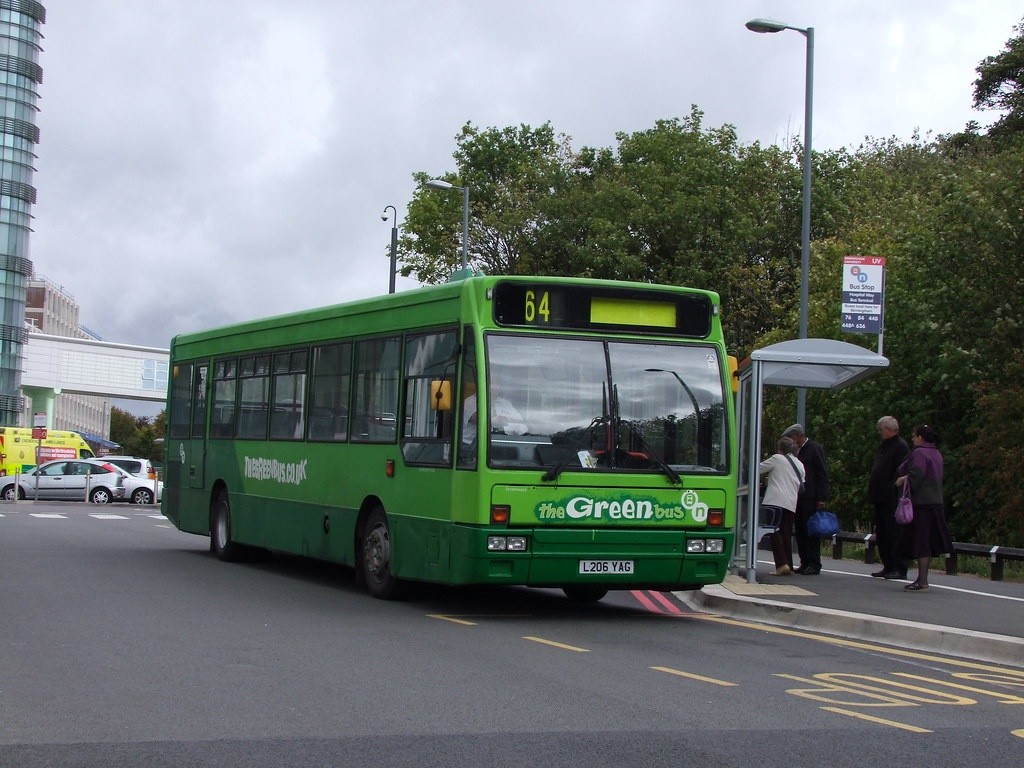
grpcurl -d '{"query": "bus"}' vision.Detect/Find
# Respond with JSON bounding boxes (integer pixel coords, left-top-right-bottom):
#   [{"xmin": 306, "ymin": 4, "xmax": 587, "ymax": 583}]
[{"xmin": 161, "ymin": 276, "xmax": 739, "ymax": 601}]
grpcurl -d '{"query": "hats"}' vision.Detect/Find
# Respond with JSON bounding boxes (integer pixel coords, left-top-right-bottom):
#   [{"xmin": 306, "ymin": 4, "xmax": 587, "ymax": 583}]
[{"xmin": 782, "ymin": 424, "xmax": 803, "ymax": 436}]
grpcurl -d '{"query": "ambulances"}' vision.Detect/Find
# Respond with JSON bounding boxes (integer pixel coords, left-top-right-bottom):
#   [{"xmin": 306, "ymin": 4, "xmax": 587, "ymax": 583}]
[{"xmin": 0, "ymin": 427, "xmax": 97, "ymax": 476}]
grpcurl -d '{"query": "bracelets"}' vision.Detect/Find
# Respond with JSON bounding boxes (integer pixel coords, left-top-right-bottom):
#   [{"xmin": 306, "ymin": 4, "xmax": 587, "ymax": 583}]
[{"xmin": 901, "ymin": 477, "xmax": 904, "ymax": 479}]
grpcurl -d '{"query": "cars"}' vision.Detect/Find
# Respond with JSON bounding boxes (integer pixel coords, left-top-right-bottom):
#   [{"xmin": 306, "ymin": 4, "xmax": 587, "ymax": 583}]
[
  {"xmin": 469, "ymin": 432, "xmax": 586, "ymax": 472},
  {"xmin": 0, "ymin": 459, "xmax": 125, "ymax": 505},
  {"xmin": 651, "ymin": 463, "xmax": 718, "ymax": 473},
  {"xmin": 44, "ymin": 461, "xmax": 164, "ymax": 504},
  {"xmin": 88, "ymin": 456, "xmax": 156, "ymax": 480}
]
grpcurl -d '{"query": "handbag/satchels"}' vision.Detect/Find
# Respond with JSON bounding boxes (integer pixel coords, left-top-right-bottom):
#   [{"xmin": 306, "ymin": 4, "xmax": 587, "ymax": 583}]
[
  {"xmin": 807, "ymin": 510, "xmax": 839, "ymax": 537},
  {"xmin": 894, "ymin": 475, "xmax": 913, "ymax": 524}
]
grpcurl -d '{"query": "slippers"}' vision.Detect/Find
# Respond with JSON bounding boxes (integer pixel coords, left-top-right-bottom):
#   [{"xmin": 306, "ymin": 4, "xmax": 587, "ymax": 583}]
[{"xmin": 903, "ymin": 582, "xmax": 930, "ymax": 592}]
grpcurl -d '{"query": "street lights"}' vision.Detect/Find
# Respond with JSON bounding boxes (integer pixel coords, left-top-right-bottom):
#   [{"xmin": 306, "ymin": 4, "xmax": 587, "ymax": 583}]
[
  {"xmin": 644, "ymin": 368, "xmax": 702, "ymax": 442},
  {"xmin": 744, "ymin": 17, "xmax": 814, "ymax": 442},
  {"xmin": 101, "ymin": 401, "xmax": 108, "ymax": 439},
  {"xmin": 381, "ymin": 205, "xmax": 398, "ymax": 293},
  {"xmin": 425, "ymin": 180, "xmax": 467, "ymax": 271}
]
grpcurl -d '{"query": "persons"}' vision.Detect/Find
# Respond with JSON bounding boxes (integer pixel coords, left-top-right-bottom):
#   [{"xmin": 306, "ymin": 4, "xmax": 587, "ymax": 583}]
[
  {"xmin": 894, "ymin": 422, "xmax": 955, "ymax": 592},
  {"xmin": 867, "ymin": 415, "xmax": 911, "ymax": 579},
  {"xmin": 782, "ymin": 423, "xmax": 829, "ymax": 575},
  {"xmin": 758, "ymin": 437, "xmax": 806, "ymax": 575},
  {"xmin": 462, "ymin": 375, "xmax": 528, "ymax": 445}
]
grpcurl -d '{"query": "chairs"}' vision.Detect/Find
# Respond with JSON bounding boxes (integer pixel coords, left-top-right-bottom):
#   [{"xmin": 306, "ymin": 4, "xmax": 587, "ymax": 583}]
[{"xmin": 218, "ymin": 406, "xmax": 411, "ymax": 441}]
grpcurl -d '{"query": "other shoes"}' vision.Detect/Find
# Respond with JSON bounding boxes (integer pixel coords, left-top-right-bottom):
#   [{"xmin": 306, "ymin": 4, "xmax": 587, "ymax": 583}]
[
  {"xmin": 769, "ymin": 564, "xmax": 789, "ymax": 575},
  {"xmin": 787, "ymin": 570, "xmax": 794, "ymax": 576},
  {"xmin": 884, "ymin": 568, "xmax": 907, "ymax": 580},
  {"xmin": 801, "ymin": 565, "xmax": 821, "ymax": 575},
  {"xmin": 871, "ymin": 567, "xmax": 890, "ymax": 577},
  {"xmin": 793, "ymin": 564, "xmax": 808, "ymax": 573}
]
